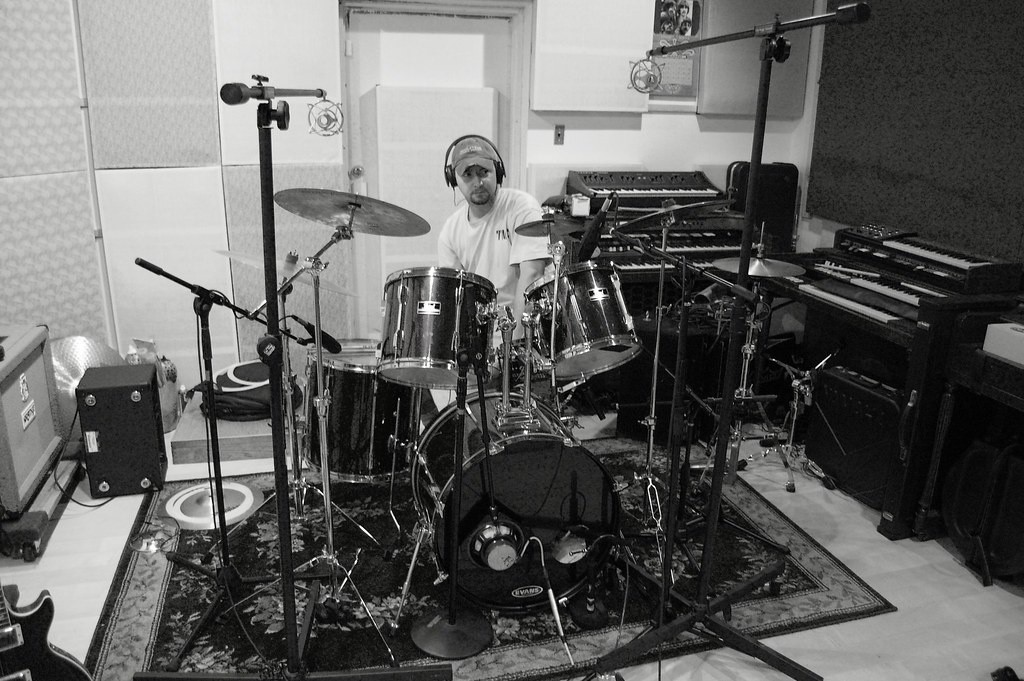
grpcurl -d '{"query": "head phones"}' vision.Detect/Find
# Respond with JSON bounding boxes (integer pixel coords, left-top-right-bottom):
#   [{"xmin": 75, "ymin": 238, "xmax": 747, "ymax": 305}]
[{"xmin": 443, "ymin": 135, "xmax": 506, "ymax": 190}]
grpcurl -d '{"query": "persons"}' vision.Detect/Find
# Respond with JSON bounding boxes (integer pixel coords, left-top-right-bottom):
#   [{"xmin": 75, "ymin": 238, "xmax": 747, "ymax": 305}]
[
  {"xmin": 661, "ymin": 0, "xmax": 692, "ymax": 35},
  {"xmin": 438, "ymin": 133, "xmax": 561, "ymax": 420}
]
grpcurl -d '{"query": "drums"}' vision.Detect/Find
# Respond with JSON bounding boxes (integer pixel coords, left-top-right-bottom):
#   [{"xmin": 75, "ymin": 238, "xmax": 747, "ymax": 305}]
[
  {"xmin": 301, "ymin": 338, "xmax": 418, "ymax": 485},
  {"xmin": 491, "ymin": 260, "xmax": 644, "ymax": 400},
  {"xmin": 410, "ymin": 389, "xmax": 622, "ymax": 614},
  {"xmin": 376, "ymin": 266, "xmax": 498, "ymax": 389}
]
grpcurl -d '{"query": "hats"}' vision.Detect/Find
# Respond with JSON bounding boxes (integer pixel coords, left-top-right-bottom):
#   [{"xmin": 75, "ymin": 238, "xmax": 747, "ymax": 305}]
[{"xmin": 451, "ymin": 137, "xmax": 503, "ymax": 176}]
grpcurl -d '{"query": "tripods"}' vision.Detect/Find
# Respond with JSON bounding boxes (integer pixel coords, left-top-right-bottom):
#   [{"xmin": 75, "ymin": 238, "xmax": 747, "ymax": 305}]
[
  {"xmin": 135, "ymin": 206, "xmax": 401, "ymax": 673},
  {"xmin": 583, "ymin": 219, "xmax": 836, "ymax": 648}
]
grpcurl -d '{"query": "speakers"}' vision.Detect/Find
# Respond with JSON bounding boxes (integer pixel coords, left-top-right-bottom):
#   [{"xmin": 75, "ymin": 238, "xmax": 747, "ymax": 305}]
[
  {"xmin": 804, "ymin": 365, "xmax": 915, "ymax": 517},
  {"xmin": 75, "ymin": 363, "xmax": 169, "ymax": 499},
  {"xmin": 0, "ymin": 324, "xmax": 65, "ymax": 520}
]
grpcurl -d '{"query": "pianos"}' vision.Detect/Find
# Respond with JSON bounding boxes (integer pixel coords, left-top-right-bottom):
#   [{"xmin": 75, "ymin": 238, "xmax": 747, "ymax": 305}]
[
  {"xmin": 762, "ymin": 222, "xmax": 1024, "ymax": 541},
  {"xmin": 562, "ymin": 171, "xmax": 761, "ymax": 434}
]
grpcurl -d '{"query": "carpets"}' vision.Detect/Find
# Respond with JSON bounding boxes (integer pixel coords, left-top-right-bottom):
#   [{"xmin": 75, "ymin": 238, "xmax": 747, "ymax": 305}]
[{"xmin": 76, "ymin": 438, "xmax": 898, "ymax": 681}]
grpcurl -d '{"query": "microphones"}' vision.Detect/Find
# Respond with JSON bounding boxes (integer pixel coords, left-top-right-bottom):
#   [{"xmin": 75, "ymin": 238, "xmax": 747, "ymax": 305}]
[
  {"xmin": 486, "ymin": 538, "xmax": 516, "ymax": 570},
  {"xmin": 642, "ymin": 72, "xmax": 657, "ymax": 88},
  {"xmin": 578, "ymin": 192, "xmax": 615, "ymax": 262},
  {"xmin": 291, "ymin": 315, "xmax": 342, "ymax": 354},
  {"xmin": 317, "ymin": 114, "xmax": 334, "ymax": 130}
]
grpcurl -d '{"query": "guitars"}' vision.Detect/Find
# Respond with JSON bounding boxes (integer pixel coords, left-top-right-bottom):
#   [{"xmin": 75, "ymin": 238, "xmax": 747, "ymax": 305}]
[{"xmin": 0, "ymin": 574, "xmax": 94, "ymax": 681}]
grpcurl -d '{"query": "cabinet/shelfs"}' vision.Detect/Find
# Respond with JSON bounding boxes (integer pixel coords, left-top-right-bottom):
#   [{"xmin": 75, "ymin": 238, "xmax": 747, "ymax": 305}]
[{"xmin": 528, "ymin": 0, "xmax": 813, "ymax": 120}]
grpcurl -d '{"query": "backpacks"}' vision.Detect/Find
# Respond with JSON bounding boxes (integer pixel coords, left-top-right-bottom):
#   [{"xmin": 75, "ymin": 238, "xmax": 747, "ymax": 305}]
[{"xmin": 187, "ymin": 359, "xmax": 304, "ymax": 423}]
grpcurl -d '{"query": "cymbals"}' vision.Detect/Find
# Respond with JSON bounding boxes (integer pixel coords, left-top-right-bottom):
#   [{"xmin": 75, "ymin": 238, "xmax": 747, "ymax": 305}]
[
  {"xmin": 215, "ymin": 249, "xmax": 360, "ymax": 299},
  {"xmin": 515, "ymin": 218, "xmax": 585, "ymax": 238},
  {"xmin": 616, "ymin": 200, "xmax": 735, "ymax": 231},
  {"xmin": 711, "ymin": 258, "xmax": 806, "ymax": 279},
  {"xmin": 274, "ymin": 188, "xmax": 431, "ymax": 237},
  {"xmin": 158, "ymin": 481, "xmax": 265, "ymax": 529}
]
